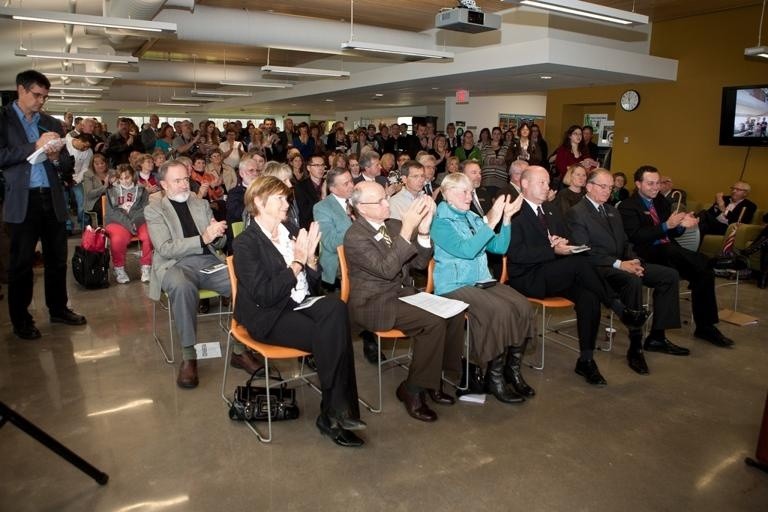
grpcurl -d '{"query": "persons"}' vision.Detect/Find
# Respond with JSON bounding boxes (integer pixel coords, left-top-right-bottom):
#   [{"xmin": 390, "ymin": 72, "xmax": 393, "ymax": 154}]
[
  {"xmin": 744, "ymin": 115, "xmax": 767, "ymax": 136},
  {"xmin": 1, "ymin": 69, "xmax": 87, "ymax": 339},
  {"xmin": 688, "ymin": 180, "xmax": 757, "ymax": 291},
  {"xmin": 59, "ymin": 108, "xmax": 733, "ymax": 448}
]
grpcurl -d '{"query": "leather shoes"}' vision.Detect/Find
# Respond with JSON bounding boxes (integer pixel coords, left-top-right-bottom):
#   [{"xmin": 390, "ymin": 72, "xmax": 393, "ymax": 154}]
[
  {"xmin": 177, "ymin": 358, "xmax": 199, "ymax": 390},
  {"xmin": 396, "ymin": 380, "xmax": 437, "ymax": 422},
  {"xmin": 626, "ymin": 349, "xmax": 649, "ymax": 374},
  {"xmin": 694, "ymin": 324, "xmax": 735, "ymax": 347},
  {"xmin": 198, "ymin": 298, "xmax": 210, "ymax": 314},
  {"xmin": 623, "ymin": 310, "xmax": 653, "ymax": 330},
  {"xmin": 230, "ymin": 352, "xmax": 271, "ymax": 376},
  {"xmin": 49, "ymin": 309, "xmax": 87, "ymax": 325},
  {"xmin": 12, "ymin": 318, "xmax": 42, "ymax": 340},
  {"xmin": 425, "ymin": 378, "xmax": 456, "ymax": 405},
  {"xmin": 364, "ymin": 341, "xmax": 386, "ymax": 366},
  {"xmin": 297, "ymin": 354, "xmax": 317, "ymax": 369},
  {"xmin": 574, "ymin": 356, "xmax": 607, "ymax": 388},
  {"xmin": 644, "ymin": 334, "xmax": 690, "ymax": 355}
]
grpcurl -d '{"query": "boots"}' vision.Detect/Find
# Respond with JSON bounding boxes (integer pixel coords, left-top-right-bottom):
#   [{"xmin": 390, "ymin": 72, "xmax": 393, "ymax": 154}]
[
  {"xmin": 484, "ymin": 345, "xmax": 526, "ymax": 404},
  {"xmin": 504, "ymin": 336, "xmax": 535, "ymax": 396}
]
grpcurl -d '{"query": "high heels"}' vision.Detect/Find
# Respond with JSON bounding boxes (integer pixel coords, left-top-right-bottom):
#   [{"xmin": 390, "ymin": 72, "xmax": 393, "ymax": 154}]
[
  {"xmin": 317, "ymin": 415, "xmax": 364, "ymax": 447},
  {"xmin": 328, "ymin": 407, "xmax": 367, "ymax": 430}
]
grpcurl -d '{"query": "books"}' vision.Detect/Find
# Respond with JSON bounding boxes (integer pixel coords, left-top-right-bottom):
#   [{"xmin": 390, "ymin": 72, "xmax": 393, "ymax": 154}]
[
  {"xmin": 25, "ymin": 137, "xmax": 69, "ymax": 166},
  {"xmin": 716, "ymin": 306, "xmax": 759, "ymax": 327}
]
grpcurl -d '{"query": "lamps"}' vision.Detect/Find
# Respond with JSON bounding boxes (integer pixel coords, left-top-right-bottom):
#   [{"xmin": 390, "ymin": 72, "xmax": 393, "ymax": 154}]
[
  {"xmin": 0, "ymin": 3, "xmax": 179, "ymax": 103},
  {"xmin": 260, "ymin": 2, "xmax": 457, "ymax": 79}
]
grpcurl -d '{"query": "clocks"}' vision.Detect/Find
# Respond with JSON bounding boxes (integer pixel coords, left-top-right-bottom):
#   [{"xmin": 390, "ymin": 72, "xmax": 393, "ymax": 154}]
[{"xmin": 620, "ymin": 89, "xmax": 640, "ymax": 112}]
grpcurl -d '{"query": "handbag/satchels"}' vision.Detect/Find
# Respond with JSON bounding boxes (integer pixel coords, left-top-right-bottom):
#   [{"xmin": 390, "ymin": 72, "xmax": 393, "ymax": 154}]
[
  {"xmin": 82, "ymin": 229, "xmax": 107, "ymax": 253},
  {"xmin": 71, "ymin": 246, "xmax": 111, "ymax": 289},
  {"xmin": 229, "ymin": 385, "xmax": 299, "ymax": 420}
]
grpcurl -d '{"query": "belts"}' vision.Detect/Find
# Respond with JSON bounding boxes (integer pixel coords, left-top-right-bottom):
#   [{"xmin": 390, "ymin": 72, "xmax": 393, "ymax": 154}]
[{"xmin": 29, "ymin": 185, "xmax": 51, "ymax": 194}]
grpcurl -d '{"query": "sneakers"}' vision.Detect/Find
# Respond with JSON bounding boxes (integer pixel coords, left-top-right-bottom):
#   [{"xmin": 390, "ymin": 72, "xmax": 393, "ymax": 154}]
[
  {"xmin": 113, "ymin": 265, "xmax": 130, "ymax": 284},
  {"xmin": 140, "ymin": 264, "xmax": 153, "ymax": 283}
]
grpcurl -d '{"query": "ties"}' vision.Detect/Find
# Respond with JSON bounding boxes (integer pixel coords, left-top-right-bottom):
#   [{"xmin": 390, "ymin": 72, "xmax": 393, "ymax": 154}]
[
  {"xmin": 425, "ymin": 183, "xmax": 433, "ymax": 197},
  {"xmin": 379, "ymin": 226, "xmax": 392, "ymax": 249},
  {"xmin": 597, "ymin": 204, "xmax": 610, "ymax": 228},
  {"xmin": 537, "ymin": 206, "xmax": 549, "ymax": 230},
  {"xmin": 649, "ymin": 202, "xmax": 668, "ymax": 243}
]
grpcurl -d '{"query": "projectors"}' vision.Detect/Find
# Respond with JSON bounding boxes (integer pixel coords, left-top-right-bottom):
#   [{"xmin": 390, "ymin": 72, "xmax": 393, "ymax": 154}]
[{"xmin": 434, "ymin": 8, "xmax": 501, "ymax": 34}]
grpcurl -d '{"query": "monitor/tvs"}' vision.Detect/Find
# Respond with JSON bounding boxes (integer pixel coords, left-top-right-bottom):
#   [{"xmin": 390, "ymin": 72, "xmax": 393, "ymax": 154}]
[{"xmin": 718, "ymin": 83, "xmax": 768, "ymax": 148}]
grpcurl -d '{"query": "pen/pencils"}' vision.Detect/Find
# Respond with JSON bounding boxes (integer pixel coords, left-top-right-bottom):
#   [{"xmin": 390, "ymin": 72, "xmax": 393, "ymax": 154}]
[{"xmin": 38, "ymin": 126, "xmax": 48, "ymax": 132}]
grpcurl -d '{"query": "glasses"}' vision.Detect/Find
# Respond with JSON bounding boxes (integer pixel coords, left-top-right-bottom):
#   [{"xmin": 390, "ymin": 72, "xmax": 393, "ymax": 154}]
[
  {"xmin": 310, "ymin": 163, "xmax": 325, "ymax": 168},
  {"xmin": 729, "ymin": 186, "xmax": 747, "ymax": 192},
  {"xmin": 29, "ymin": 89, "xmax": 49, "ymax": 102},
  {"xmin": 360, "ymin": 194, "xmax": 390, "ymax": 206},
  {"xmin": 592, "ymin": 182, "xmax": 615, "ymax": 190}
]
